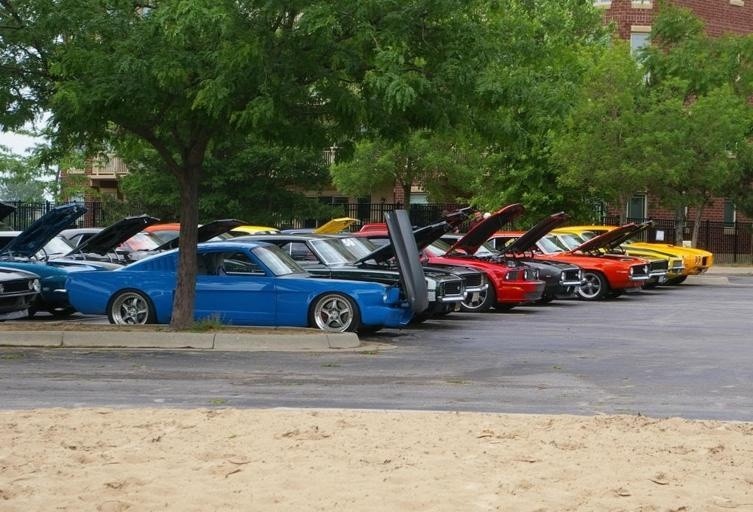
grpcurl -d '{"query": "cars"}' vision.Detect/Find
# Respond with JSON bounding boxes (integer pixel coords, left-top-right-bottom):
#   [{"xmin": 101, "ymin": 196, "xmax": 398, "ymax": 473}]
[
  {"xmin": 0, "ymin": 201, "xmax": 109, "ymax": 316},
  {"xmin": 0, "ymin": 266, "xmax": 41, "ymax": 315},
  {"xmin": 2, "ymin": 197, "xmax": 370, "ymax": 315},
  {"xmin": 64, "ymin": 210, "xmax": 430, "ymax": 338},
  {"xmin": 362, "ymin": 199, "xmax": 717, "ymax": 325}
]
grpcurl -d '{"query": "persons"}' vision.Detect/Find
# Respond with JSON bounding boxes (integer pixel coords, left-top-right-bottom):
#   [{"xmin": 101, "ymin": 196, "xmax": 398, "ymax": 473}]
[
  {"xmin": 468, "ymin": 210, "xmax": 484, "ymax": 230},
  {"xmin": 440, "ymin": 210, "xmax": 459, "ymax": 234}
]
